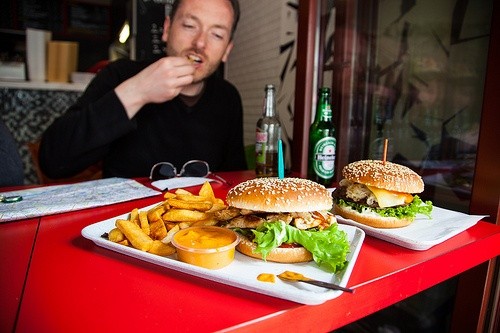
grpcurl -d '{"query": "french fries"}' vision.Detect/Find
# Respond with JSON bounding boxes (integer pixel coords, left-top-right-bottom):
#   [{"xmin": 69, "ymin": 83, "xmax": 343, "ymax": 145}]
[{"xmin": 108, "ymin": 180, "xmax": 227, "ymax": 257}]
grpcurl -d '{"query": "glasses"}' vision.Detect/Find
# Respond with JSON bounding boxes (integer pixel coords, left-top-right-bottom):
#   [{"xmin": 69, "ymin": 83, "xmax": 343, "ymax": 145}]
[{"xmin": 149, "ymin": 160, "xmax": 233, "ymax": 192}]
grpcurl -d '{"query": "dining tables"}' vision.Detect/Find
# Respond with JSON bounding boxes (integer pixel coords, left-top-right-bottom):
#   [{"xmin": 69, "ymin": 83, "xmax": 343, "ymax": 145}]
[{"xmin": 0, "ymin": 169, "xmax": 500, "ymax": 333}]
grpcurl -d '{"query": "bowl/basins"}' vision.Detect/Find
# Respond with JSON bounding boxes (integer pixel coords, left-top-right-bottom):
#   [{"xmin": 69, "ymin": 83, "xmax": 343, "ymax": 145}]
[{"xmin": 172, "ymin": 226, "xmax": 241, "ymax": 269}]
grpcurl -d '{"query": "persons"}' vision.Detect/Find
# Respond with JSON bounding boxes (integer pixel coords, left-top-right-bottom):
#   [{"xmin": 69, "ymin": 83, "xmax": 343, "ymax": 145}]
[
  {"xmin": 38, "ymin": 0, "xmax": 248, "ymax": 178},
  {"xmin": 0, "ymin": 118, "xmax": 24, "ymax": 187}
]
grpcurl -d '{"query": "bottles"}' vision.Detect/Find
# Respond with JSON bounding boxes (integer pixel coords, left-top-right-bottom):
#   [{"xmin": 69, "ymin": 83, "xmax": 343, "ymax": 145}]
[
  {"xmin": 255, "ymin": 83, "xmax": 282, "ymax": 178},
  {"xmin": 308, "ymin": 87, "xmax": 337, "ymax": 188}
]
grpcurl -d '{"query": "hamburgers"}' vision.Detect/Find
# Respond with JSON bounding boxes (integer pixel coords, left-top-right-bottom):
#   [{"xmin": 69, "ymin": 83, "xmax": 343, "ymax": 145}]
[
  {"xmin": 331, "ymin": 160, "xmax": 433, "ymax": 228},
  {"xmin": 215, "ymin": 177, "xmax": 351, "ymax": 272}
]
